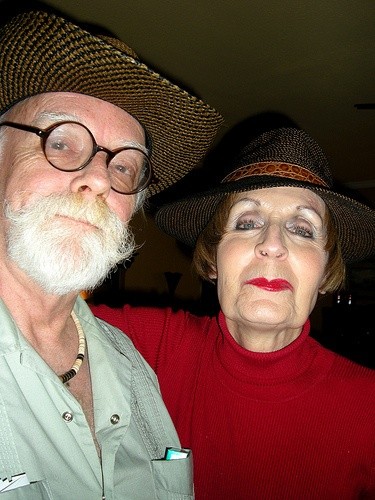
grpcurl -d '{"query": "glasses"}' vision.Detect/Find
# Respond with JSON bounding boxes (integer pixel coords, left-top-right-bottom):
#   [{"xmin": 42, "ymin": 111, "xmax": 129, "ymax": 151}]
[{"xmin": 0, "ymin": 121, "xmax": 159, "ymax": 194}]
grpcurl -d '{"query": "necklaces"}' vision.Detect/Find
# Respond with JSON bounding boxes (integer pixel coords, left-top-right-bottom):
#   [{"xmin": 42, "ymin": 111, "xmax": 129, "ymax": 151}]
[{"xmin": 52, "ymin": 307, "xmax": 85, "ymax": 384}]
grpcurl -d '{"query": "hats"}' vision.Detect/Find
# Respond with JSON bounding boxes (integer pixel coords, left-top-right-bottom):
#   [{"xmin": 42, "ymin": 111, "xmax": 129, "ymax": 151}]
[
  {"xmin": 154, "ymin": 127, "xmax": 374, "ymax": 264},
  {"xmin": 0, "ymin": 13, "xmax": 225, "ymax": 198}
]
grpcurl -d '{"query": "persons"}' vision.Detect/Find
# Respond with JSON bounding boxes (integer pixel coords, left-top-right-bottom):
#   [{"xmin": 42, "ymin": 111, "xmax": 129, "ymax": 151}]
[
  {"xmin": 85, "ymin": 127, "xmax": 374, "ymax": 500},
  {"xmin": 0, "ymin": 1, "xmax": 225, "ymax": 500}
]
